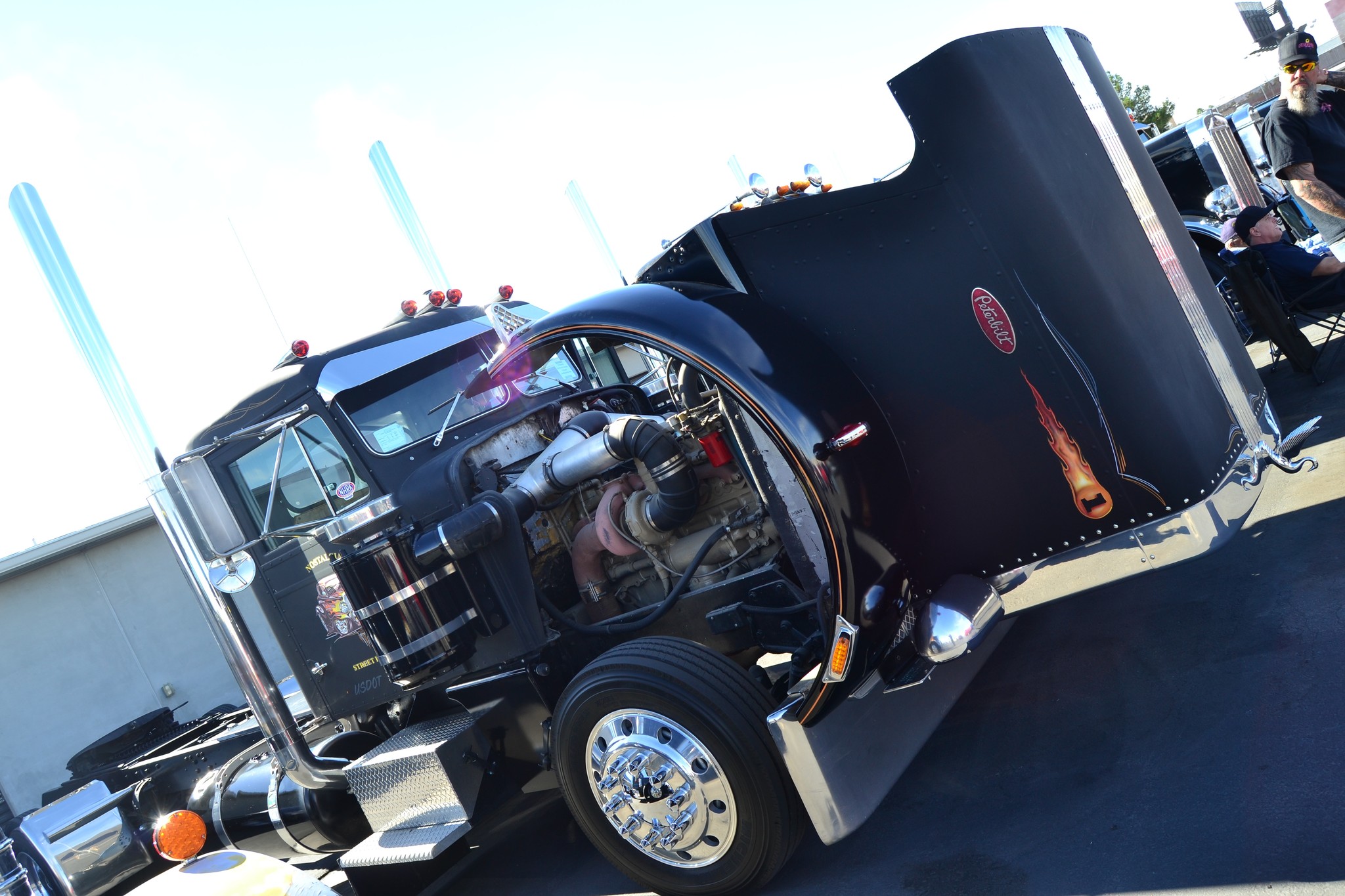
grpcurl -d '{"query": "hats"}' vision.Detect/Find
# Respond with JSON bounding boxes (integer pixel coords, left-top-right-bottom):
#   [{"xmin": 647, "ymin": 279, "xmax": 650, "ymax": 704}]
[
  {"xmin": 1234, "ymin": 202, "xmax": 1276, "ymax": 242},
  {"xmin": 1220, "ymin": 218, "xmax": 1238, "ymax": 243},
  {"xmin": 1278, "ymin": 32, "xmax": 1319, "ymax": 67}
]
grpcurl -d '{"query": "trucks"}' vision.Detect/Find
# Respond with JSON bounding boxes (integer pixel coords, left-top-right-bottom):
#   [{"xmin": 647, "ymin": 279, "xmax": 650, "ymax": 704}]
[{"xmin": 0, "ymin": 23, "xmax": 1329, "ymax": 895}]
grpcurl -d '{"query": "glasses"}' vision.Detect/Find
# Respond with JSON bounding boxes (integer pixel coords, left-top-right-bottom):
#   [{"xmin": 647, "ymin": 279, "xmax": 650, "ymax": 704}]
[{"xmin": 1279, "ymin": 62, "xmax": 1319, "ymax": 74}]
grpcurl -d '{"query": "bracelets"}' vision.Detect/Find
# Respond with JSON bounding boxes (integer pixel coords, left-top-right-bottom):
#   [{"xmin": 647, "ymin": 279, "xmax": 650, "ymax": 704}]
[{"xmin": 1320, "ymin": 68, "xmax": 1329, "ymax": 85}]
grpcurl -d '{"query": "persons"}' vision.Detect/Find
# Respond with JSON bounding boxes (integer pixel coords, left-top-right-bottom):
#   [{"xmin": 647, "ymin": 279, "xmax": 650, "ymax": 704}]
[
  {"xmin": 440, "ymin": 386, "xmax": 482, "ymax": 423},
  {"xmin": 1234, "ymin": 200, "xmax": 1345, "ymax": 310},
  {"xmin": 1221, "ymin": 217, "xmax": 1334, "ymax": 257},
  {"xmin": 1258, "ymin": 31, "xmax": 1345, "ymax": 267}
]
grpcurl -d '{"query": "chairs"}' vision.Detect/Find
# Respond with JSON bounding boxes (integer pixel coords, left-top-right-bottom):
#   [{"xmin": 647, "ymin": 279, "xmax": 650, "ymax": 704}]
[{"xmin": 1215, "ymin": 249, "xmax": 1345, "ymax": 386}]
